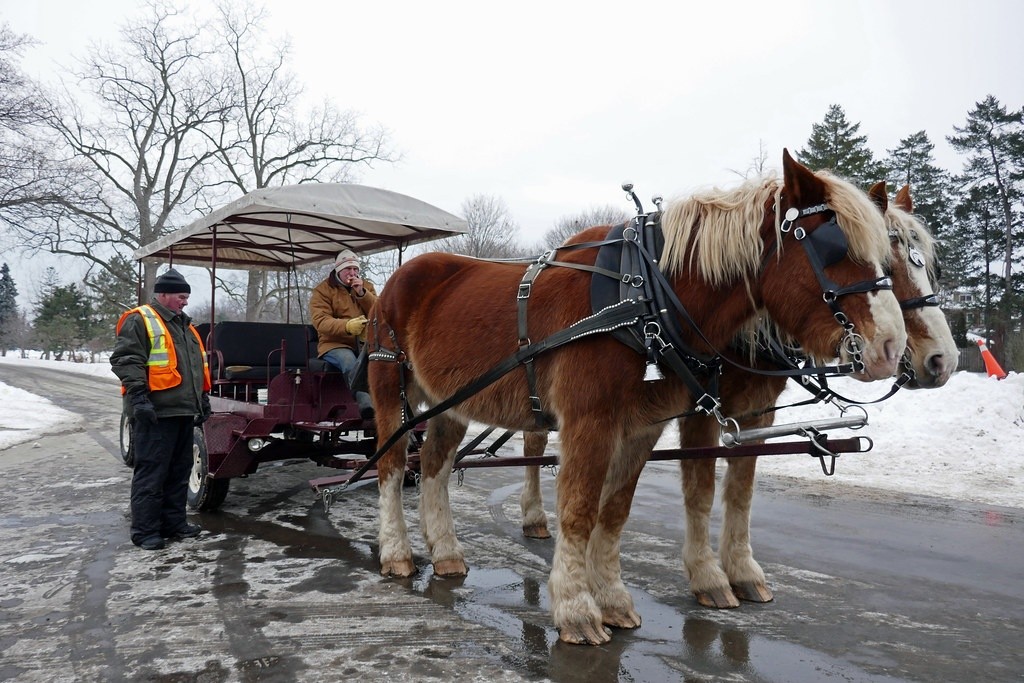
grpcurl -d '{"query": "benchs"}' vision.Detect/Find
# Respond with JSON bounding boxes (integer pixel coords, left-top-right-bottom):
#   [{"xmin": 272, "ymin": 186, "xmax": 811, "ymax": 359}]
[{"xmin": 207, "ymin": 320, "xmax": 322, "ymax": 403}]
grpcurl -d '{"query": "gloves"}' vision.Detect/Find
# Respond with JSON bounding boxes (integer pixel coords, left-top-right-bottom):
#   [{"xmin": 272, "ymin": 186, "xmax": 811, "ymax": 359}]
[
  {"xmin": 134, "ymin": 402, "xmax": 158, "ymax": 425},
  {"xmin": 346, "ymin": 314, "xmax": 367, "ymax": 341},
  {"xmin": 193, "ymin": 396, "xmax": 211, "ymax": 426}
]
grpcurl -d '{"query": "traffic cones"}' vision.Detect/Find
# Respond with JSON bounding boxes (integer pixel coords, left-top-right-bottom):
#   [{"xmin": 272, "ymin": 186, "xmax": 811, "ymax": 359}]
[{"xmin": 978, "ymin": 340, "xmax": 1006, "ymax": 378}]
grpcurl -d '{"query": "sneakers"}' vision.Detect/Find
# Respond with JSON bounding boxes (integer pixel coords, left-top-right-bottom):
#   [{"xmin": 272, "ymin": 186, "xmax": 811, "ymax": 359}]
[
  {"xmin": 175, "ymin": 525, "xmax": 201, "ymax": 538},
  {"xmin": 362, "ymin": 405, "xmax": 375, "ymax": 420},
  {"xmin": 142, "ymin": 536, "xmax": 164, "ymax": 550}
]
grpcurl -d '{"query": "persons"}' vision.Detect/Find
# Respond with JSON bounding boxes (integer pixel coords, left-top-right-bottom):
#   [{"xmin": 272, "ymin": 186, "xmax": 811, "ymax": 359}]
[
  {"xmin": 308, "ymin": 249, "xmax": 382, "ymax": 419},
  {"xmin": 109, "ymin": 268, "xmax": 213, "ymax": 550}
]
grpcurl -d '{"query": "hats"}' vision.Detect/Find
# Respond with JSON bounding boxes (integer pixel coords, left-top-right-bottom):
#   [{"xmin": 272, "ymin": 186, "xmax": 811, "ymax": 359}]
[
  {"xmin": 154, "ymin": 268, "xmax": 191, "ymax": 293},
  {"xmin": 335, "ymin": 250, "xmax": 360, "ymax": 272}
]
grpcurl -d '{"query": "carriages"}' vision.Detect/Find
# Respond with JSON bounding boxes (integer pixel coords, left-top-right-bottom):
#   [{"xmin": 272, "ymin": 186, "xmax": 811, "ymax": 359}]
[{"xmin": 132, "ymin": 147, "xmax": 963, "ymax": 646}]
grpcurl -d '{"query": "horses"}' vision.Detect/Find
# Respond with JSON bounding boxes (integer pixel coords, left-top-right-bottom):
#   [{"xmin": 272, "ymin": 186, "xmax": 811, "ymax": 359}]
[{"xmin": 365, "ymin": 148, "xmax": 961, "ymax": 647}]
